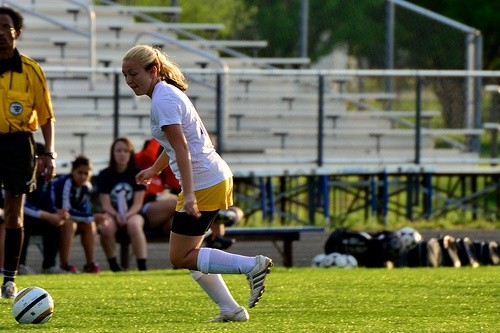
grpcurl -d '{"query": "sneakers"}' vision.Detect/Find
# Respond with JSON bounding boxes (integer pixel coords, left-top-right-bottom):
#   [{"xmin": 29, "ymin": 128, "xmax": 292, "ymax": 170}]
[
  {"xmin": 246, "ymin": 254, "xmax": 273, "ymax": 308},
  {"xmin": 1, "ymin": 281, "xmax": 17, "ymax": 298},
  {"xmin": 213, "ymin": 306, "xmax": 249, "ymax": 322}
]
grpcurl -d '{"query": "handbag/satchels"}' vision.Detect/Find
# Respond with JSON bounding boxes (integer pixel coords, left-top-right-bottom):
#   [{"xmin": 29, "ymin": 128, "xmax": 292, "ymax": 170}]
[{"xmin": 323, "ymin": 225, "xmax": 500, "ymax": 268}]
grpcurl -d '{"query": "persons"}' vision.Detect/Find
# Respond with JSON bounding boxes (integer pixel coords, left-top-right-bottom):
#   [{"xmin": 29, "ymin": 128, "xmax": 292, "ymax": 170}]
[
  {"xmin": 91, "ymin": 138, "xmax": 148, "ymax": 271},
  {"xmin": 0, "ymin": 7, "xmax": 56, "ymax": 299},
  {"xmin": 55, "ymin": 157, "xmax": 110, "ymax": 274},
  {"xmin": 0, "ymin": 143, "xmax": 70, "ymax": 274},
  {"xmin": 122, "ymin": 44, "xmax": 272, "ymax": 322},
  {"xmin": 135, "ymin": 140, "xmax": 242, "ymax": 250}
]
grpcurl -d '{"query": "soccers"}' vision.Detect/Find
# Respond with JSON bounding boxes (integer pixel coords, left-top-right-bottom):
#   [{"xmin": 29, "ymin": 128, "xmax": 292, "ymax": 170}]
[
  {"xmin": 342, "ymin": 254, "xmax": 359, "ymax": 268},
  {"xmin": 324, "ymin": 253, "xmax": 344, "ymax": 268},
  {"xmin": 310, "ymin": 253, "xmax": 327, "ymax": 268},
  {"xmin": 396, "ymin": 227, "xmax": 423, "ymax": 250},
  {"xmin": 11, "ymin": 286, "xmax": 54, "ymax": 324}
]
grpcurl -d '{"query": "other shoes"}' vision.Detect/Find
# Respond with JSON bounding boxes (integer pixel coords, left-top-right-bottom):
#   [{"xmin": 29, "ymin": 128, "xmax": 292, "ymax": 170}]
[
  {"xmin": 83, "ymin": 261, "xmax": 100, "ymax": 273},
  {"xmin": 18, "ymin": 264, "xmax": 33, "ymax": 275},
  {"xmin": 59, "ymin": 264, "xmax": 79, "ymax": 273},
  {"xmin": 205, "ymin": 233, "xmax": 236, "ymax": 251},
  {"xmin": 214, "ymin": 210, "xmax": 234, "ymax": 223},
  {"xmin": 43, "ymin": 265, "xmax": 66, "ymax": 273}
]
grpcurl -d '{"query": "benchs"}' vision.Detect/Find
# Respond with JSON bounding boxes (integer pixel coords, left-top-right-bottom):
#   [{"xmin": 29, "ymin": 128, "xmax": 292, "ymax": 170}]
[
  {"xmin": 0, "ymin": 1, "xmax": 500, "ymax": 171},
  {"xmin": 223, "ymin": 227, "xmax": 323, "ymax": 268}
]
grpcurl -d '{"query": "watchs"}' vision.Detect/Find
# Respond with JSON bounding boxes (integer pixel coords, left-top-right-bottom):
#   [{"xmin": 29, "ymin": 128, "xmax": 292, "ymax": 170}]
[{"xmin": 44, "ymin": 152, "xmax": 57, "ymax": 159}]
